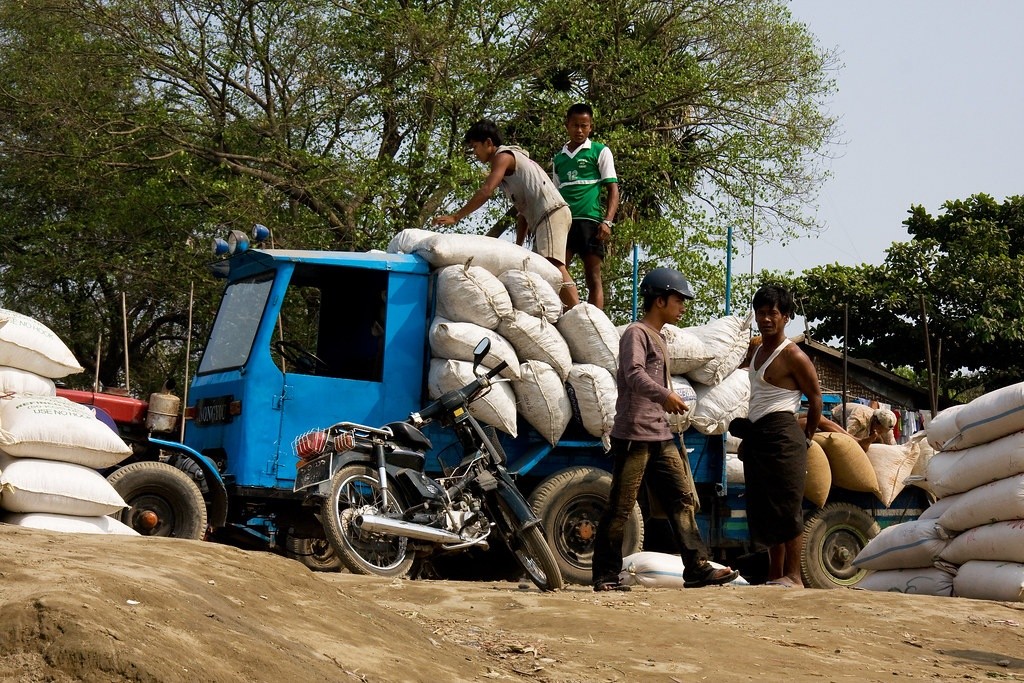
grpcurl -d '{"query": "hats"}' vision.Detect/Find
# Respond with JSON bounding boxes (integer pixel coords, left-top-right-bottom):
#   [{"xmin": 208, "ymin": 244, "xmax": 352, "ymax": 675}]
[{"xmin": 873, "ymin": 409, "xmax": 897, "ymax": 430}]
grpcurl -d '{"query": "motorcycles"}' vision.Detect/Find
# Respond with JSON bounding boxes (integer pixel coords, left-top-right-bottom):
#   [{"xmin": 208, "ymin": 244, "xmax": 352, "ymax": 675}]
[{"xmin": 288, "ymin": 338, "xmax": 567, "ymax": 594}]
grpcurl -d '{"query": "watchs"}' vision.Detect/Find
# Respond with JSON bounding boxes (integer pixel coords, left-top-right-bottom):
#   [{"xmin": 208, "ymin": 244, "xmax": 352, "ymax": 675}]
[{"xmin": 602, "ymin": 219, "xmax": 613, "ymax": 228}]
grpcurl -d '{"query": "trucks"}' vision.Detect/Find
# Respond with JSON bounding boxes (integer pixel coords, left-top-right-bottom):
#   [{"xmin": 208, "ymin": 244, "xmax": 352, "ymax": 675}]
[
  {"xmin": 691, "ymin": 398, "xmax": 932, "ymax": 591},
  {"xmin": 170, "ymin": 224, "xmax": 729, "ymax": 587}
]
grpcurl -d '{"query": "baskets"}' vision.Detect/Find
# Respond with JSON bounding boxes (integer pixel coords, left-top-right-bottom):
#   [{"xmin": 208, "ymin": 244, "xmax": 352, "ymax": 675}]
[{"xmin": 437, "ymin": 426, "xmax": 508, "ymax": 478}]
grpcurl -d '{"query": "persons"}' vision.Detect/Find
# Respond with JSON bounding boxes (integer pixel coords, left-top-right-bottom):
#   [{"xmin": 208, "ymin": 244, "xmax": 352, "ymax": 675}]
[
  {"xmin": 792, "ymin": 402, "xmax": 898, "ymax": 453},
  {"xmin": 431, "ymin": 118, "xmax": 580, "ymax": 309},
  {"xmin": 551, "ymin": 102, "xmax": 620, "ymax": 315},
  {"xmin": 591, "ymin": 267, "xmax": 740, "ymax": 592},
  {"xmin": 727, "ymin": 284, "xmax": 823, "ymax": 589}
]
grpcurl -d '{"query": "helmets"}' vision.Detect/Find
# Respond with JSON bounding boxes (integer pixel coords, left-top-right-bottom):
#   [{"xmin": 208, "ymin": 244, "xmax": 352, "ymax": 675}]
[{"xmin": 640, "ymin": 267, "xmax": 693, "ymax": 300}]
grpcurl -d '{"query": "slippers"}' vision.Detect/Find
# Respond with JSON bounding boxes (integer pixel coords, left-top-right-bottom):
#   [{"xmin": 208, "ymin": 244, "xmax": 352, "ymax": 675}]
[
  {"xmin": 766, "ymin": 573, "xmax": 804, "ymax": 589},
  {"xmin": 683, "ymin": 566, "xmax": 739, "ymax": 588},
  {"xmin": 593, "ymin": 573, "xmax": 632, "ymax": 591}
]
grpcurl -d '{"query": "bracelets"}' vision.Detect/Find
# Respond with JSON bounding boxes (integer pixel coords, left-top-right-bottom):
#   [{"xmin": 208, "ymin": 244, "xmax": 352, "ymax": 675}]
[{"xmin": 806, "ymin": 438, "xmax": 814, "ymax": 447}]
